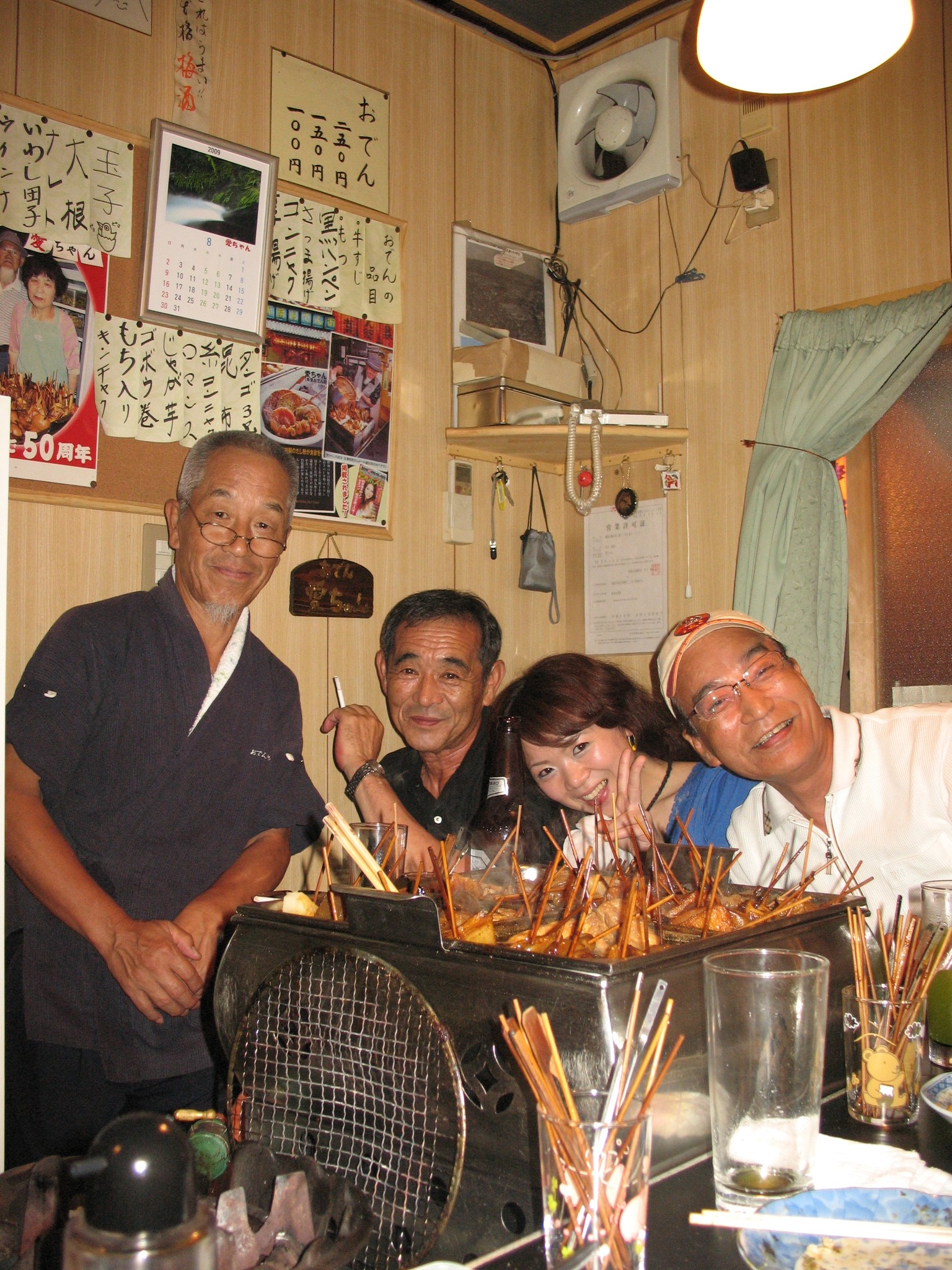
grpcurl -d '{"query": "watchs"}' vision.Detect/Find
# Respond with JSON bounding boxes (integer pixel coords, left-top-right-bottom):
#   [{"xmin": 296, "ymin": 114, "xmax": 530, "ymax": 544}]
[{"xmin": 344, "ymin": 759, "xmax": 385, "ymax": 803}]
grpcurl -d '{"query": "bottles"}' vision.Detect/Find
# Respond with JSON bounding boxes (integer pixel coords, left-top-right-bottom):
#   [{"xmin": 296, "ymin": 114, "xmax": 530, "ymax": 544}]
[
  {"xmin": 58, "ymin": 1111, "xmax": 217, "ymax": 1270},
  {"xmin": 470, "ymin": 716, "xmax": 539, "ymax": 872}
]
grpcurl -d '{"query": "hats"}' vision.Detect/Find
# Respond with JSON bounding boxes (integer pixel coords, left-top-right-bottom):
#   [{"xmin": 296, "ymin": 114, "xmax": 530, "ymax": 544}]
[{"xmin": 655, "ymin": 612, "xmax": 789, "ymax": 721}]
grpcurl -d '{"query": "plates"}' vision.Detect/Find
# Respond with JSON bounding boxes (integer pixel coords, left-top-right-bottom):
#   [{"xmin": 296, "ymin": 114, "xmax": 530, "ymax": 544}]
[
  {"xmin": 736, "ymin": 1187, "xmax": 952, "ymax": 1270},
  {"xmin": 919, "ymin": 1071, "xmax": 952, "ymax": 1123}
]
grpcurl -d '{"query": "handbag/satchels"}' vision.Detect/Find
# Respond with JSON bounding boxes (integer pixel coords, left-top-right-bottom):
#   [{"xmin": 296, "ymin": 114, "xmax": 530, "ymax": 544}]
[
  {"xmin": 290, "ymin": 533, "xmax": 374, "ymax": 618},
  {"xmin": 519, "ymin": 531, "xmax": 556, "ymax": 594}
]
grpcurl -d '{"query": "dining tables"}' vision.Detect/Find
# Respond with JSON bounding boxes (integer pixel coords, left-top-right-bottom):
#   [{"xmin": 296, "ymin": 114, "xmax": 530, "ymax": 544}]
[{"xmin": 475, "ymin": 1054, "xmax": 952, "ymax": 1270}]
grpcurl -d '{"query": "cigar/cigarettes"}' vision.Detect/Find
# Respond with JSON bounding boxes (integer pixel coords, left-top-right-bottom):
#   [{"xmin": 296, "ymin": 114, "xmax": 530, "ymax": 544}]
[{"xmin": 334, "ymin": 677, "xmax": 346, "ymax": 709}]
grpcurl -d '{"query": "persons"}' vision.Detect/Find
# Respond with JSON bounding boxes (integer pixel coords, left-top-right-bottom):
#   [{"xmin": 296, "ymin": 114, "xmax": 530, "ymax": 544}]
[
  {"xmin": 656, "ymin": 611, "xmax": 952, "ymax": 972},
  {"xmin": 488, "ymin": 652, "xmax": 766, "ymax": 846},
  {"xmin": 316, "ymin": 588, "xmax": 560, "ymax": 878},
  {"xmin": 0, "ymin": 232, "xmax": 81, "ymax": 412},
  {"xmin": 5, "ymin": 431, "xmax": 311, "ymax": 1162},
  {"xmin": 329, "ymin": 353, "xmax": 385, "ymax": 523}
]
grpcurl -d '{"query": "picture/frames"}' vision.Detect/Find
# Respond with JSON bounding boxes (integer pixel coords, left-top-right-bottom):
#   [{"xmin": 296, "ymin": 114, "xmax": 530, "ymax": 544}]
[
  {"xmin": 450, "ymin": 223, "xmax": 556, "ymax": 355},
  {"xmin": 137, "ymin": 118, "xmax": 280, "ymax": 343}
]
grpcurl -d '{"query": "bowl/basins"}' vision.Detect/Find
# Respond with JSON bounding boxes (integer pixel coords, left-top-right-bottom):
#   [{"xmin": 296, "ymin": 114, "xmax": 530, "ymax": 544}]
[{"xmin": 259, "ymin": 388, "xmax": 325, "ymax": 445}]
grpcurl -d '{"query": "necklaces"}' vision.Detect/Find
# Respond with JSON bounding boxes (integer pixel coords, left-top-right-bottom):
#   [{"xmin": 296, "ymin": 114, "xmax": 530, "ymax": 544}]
[{"xmin": 646, "ymin": 759, "xmax": 676, "ymax": 814}]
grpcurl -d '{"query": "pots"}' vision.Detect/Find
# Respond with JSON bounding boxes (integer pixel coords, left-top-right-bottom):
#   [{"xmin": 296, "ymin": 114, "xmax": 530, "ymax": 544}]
[{"xmin": 331, "ymin": 376, "xmax": 360, "ymax": 411}]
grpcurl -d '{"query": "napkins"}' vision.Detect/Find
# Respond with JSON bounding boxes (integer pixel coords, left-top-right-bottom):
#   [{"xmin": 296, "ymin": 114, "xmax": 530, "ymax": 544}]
[{"xmin": 813, "ymin": 1131, "xmax": 952, "ymax": 1196}]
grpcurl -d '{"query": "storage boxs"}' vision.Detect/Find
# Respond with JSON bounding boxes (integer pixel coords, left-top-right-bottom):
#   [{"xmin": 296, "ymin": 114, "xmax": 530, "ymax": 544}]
[{"xmin": 453, "ymin": 337, "xmax": 582, "ymax": 428}]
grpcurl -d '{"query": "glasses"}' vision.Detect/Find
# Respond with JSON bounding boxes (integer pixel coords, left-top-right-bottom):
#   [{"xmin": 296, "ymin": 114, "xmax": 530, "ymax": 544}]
[
  {"xmin": 683, "ymin": 651, "xmax": 789, "ymax": 727},
  {"xmin": 184, "ymin": 498, "xmax": 288, "ymax": 560}
]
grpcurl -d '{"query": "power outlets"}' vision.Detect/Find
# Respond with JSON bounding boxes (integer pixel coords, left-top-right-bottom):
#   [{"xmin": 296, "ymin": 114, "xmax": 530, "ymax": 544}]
[{"xmin": 746, "ymin": 158, "xmax": 780, "ymax": 227}]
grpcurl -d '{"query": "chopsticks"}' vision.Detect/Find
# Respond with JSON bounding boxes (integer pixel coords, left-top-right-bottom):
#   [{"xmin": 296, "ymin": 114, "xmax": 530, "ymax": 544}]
[{"xmin": 688, "ymin": 1207, "xmax": 952, "ymax": 1245}]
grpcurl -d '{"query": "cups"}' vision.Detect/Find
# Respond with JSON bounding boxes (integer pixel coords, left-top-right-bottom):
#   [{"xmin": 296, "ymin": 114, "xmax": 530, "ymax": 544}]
[
  {"xmin": 349, "ymin": 822, "xmax": 408, "ymax": 888},
  {"xmin": 703, "ymin": 948, "xmax": 830, "ymax": 1213},
  {"xmin": 842, "ymin": 983, "xmax": 928, "ymax": 1127},
  {"xmin": 402, "ymin": 872, "xmax": 433, "ymax": 881},
  {"xmin": 537, "ymin": 1090, "xmax": 653, "ymax": 1270},
  {"xmin": 920, "ymin": 879, "xmax": 952, "ymax": 1070}
]
